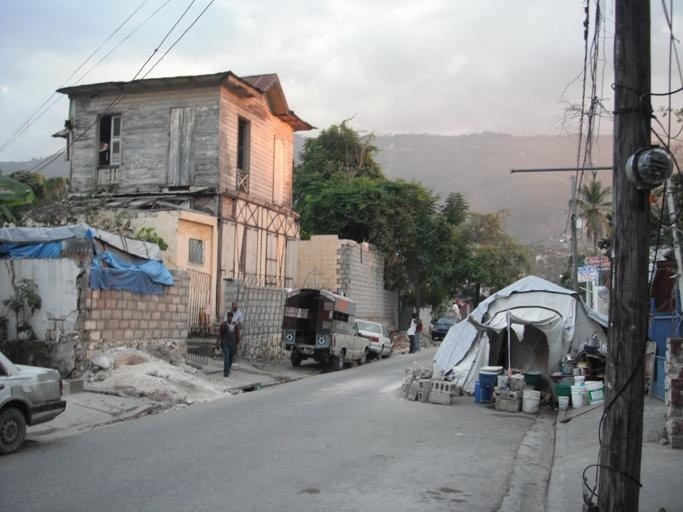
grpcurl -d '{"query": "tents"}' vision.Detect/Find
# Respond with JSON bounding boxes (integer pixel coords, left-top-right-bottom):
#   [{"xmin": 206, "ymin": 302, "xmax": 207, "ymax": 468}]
[{"xmin": 429, "ymin": 275, "xmax": 608, "ymax": 393}]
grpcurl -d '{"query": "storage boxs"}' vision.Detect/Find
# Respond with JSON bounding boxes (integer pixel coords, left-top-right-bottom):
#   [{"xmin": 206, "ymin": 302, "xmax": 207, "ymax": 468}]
[{"xmin": 474, "ymin": 364, "xmax": 525, "ymax": 413}]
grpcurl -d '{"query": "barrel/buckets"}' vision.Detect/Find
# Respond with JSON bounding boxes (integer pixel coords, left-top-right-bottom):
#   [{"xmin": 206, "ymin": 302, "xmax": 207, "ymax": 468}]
[
  {"xmin": 559, "ymin": 396, "xmax": 569, "ymax": 409},
  {"xmin": 522, "ymin": 390, "xmax": 541, "ymax": 413},
  {"xmin": 475, "ymin": 381, "xmax": 480, "ymax": 402},
  {"xmin": 571, "ymin": 386, "xmax": 586, "ymax": 408},
  {"xmin": 498, "ymin": 375, "xmax": 508, "ymax": 387},
  {"xmin": 585, "ymin": 381, "xmax": 604, "ymax": 405},
  {"xmin": 556, "ymin": 384, "xmax": 571, "ymax": 395},
  {"xmin": 574, "ymin": 376, "xmax": 586, "ymax": 387}
]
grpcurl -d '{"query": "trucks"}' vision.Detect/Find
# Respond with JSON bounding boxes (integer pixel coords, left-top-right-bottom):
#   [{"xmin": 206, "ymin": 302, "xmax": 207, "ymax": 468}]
[{"xmin": 280, "ymin": 286, "xmax": 370, "ymax": 372}]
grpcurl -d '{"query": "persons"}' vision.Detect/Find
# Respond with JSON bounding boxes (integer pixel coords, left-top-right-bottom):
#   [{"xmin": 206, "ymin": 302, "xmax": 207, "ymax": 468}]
[
  {"xmin": 429, "ymin": 320, "xmax": 434, "ymax": 335},
  {"xmin": 408, "ymin": 312, "xmax": 421, "ymax": 355},
  {"xmin": 223, "ymin": 300, "xmax": 243, "ymax": 336},
  {"xmin": 216, "ymin": 311, "xmax": 240, "ymax": 378},
  {"xmin": 408, "ymin": 312, "xmax": 422, "ymax": 351}
]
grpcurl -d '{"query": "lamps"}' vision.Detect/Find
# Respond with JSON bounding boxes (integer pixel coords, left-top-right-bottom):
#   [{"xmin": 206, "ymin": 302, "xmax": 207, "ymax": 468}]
[{"xmin": 625, "ymin": 142, "xmax": 674, "ymax": 207}]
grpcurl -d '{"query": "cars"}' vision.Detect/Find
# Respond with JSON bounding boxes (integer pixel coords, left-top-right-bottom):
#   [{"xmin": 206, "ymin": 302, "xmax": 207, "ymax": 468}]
[
  {"xmin": 354, "ymin": 319, "xmax": 395, "ymax": 361},
  {"xmin": 0, "ymin": 350, "xmax": 68, "ymax": 453},
  {"xmin": 431, "ymin": 316, "xmax": 460, "ymax": 340}
]
